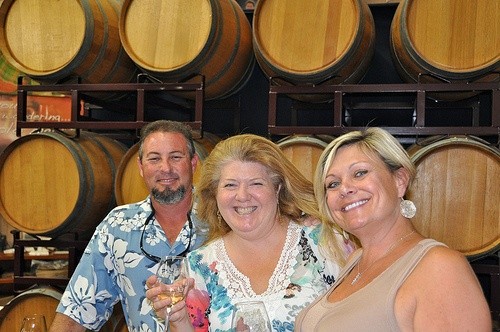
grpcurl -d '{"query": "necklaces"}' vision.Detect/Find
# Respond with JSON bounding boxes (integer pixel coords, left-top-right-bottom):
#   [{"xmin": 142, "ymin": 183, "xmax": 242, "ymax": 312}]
[{"xmin": 352, "ymin": 230, "xmax": 417, "ymax": 284}]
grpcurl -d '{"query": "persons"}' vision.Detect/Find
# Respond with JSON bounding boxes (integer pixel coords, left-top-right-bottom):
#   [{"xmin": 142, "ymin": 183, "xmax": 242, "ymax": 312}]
[
  {"xmin": 49, "ymin": 121, "xmax": 209, "ymax": 332},
  {"xmin": 147, "ymin": 135, "xmax": 354, "ymax": 332},
  {"xmin": 294, "ymin": 127, "xmax": 492, "ymax": 332}
]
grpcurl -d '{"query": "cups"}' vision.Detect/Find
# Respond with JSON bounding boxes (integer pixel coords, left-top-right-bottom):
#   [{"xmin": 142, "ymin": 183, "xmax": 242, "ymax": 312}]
[
  {"xmin": 231, "ymin": 301, "xmax": 272, "ymax": 332},
  {"xmin": 19, "ymin": 313, "xmax": 47, "ymax": 332}
]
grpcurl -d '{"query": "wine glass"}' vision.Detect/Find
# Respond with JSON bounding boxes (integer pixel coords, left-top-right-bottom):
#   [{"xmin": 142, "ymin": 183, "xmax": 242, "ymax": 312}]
[{"xmin": 154, "ymin": 256, "xmax": 190, "ymax": 332}]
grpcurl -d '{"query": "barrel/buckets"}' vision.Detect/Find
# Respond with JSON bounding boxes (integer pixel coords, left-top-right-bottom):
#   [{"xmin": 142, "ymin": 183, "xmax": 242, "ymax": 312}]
[
  {"xmin": 404, "ymin": 134, "xmax": 500, "ymax": 263},
  {"xmin": 115, "ymin": 130, "xmax": 226, "ymax": 246},
  {"xmin": 252, "ymin": 0, "xmax": 375, "ymax": 103},
  {"xmin": 389, "ymin": 0, "xmax": 500, "ymax": 102},
  {"xmin": 0, "ymin": 283, "xmax": 65, "ymax": 332},
  {"xmin": 0, "ymin": 0, "xmax": 147, "ymax": 103},
  {"xmin": 119, "ymin": 0, "xmax": 256, "ymax": 102},
  {"xmin": 0, "ymin": 128, "xmax": 131, "ymax": 241},
  {"xmin": 274, "ymin": 134, "xmax": 337, "ymax": 186}
]
